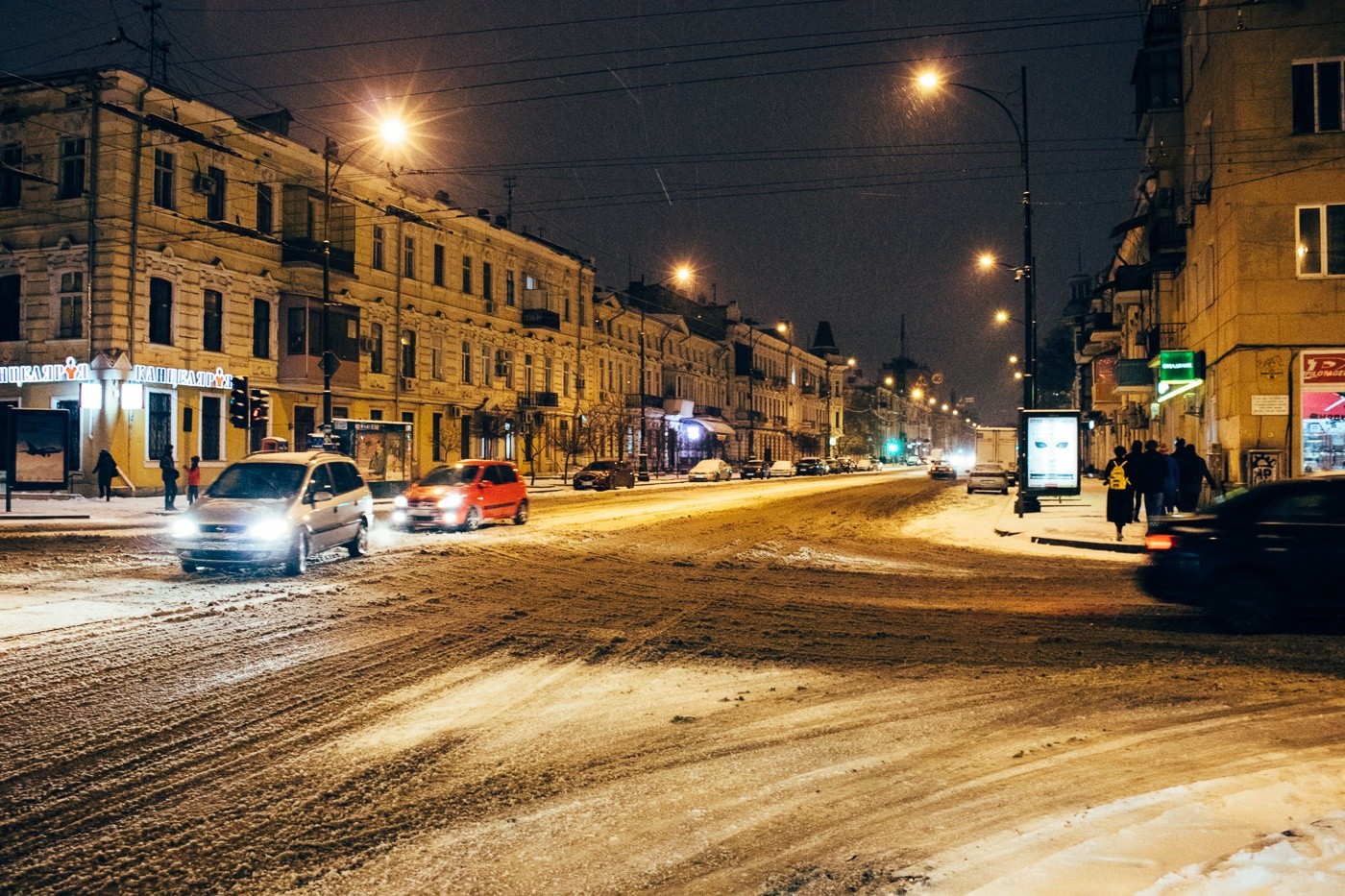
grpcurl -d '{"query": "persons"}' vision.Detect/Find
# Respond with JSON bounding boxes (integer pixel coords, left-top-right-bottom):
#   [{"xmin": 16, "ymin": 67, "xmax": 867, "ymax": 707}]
[
  {"xmin": 183, "ymin": 456, "xmax": 200, "ymax": 506},
  {"xmin": 1105, "ymin": 439, "xmax": 1217, "ymax": 541},
  {"xmin": 160, "ymin": 444, "xmax": 178, "ymax": 510},
  {"xmin": 92, "ymin": 449, "xmax": 115, "ymax": 501}
]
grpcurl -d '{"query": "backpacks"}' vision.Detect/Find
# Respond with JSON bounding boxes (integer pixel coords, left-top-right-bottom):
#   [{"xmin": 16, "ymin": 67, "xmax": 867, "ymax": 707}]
[{"xmin": 1109, "ymin": 460, "xmax": 1131, "ymax": 489}]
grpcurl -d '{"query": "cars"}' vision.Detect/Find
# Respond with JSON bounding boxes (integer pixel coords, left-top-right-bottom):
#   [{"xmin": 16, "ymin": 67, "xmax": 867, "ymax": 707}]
[
  {"xmin": 906, "ymin": 455, "xmax": 931, "ymax": 466},
  {"xmin": 930, "ymin": 460, "xmax": 958, "ymax": 479},
  {"xmin": 573, "ymin": 459, "xmax": 636, "ymax": 492},
  {"xmin": 1134, "ymin": 471, "xmax": 1345, "ymax": 636},
  {"xmin": 770, "ymin": 460, "xmax": 797, "ymax": 477},
  {"xmin": 965, "ymin": 462, "xmax": 1010, "ymax": 495},
  {"xmin": 740, "ymin": 459, "xmax": 771, "ymax": 480},
  {"xmin": 393, "ymin": 459, "xmax": 530, "ymax": 532},
  {"xmin": 688, "ymin": 459, "xmax": 733, "ymax": 482},
  {"xmin": 795, "ymin": 456, "xmax": 883, "ymax": 477}
]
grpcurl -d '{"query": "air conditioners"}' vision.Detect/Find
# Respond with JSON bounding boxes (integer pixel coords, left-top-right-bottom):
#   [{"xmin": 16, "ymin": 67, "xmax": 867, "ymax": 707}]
[
  {"xmin": 1182, "ymin": 392, "xmax": 1196, "ymax": 412},
  {"xmin": 451, "ymin": 406, "xmax": 463, "ymax": 419},
  {"xmin": 579, "ymin": 378, "xmax": 585, "ymax": 387},
  {"xmin": 1117, "ymin": 401, "xmax": 1145, "ymax": 428},
  {"xmin": 1174, "ymin": 204, "xmax": 1191, "ymax": 228},
  {"xmin": 360, "ymin": 337, "xmax": 376, "ymax": 352},
  {"xmin": 601, "ymin": 391, "xmax": 609, "ymax": 400},
  {"xmin": 499, "ymin": 350, "xmax": 509, "ymax": 361},
  {"xmin": 400, "ymin": 378, "xmax": 415, "ymax": 392},
  {"xmin": 498, "ymin": 364, "xmax": 508, "ymax": 376},
  {"xmin": 560, "ymin": 420, "xmax": 569, "ymax": 429},
  {"xmin": 194, "ymin": 172, "xmax": 217, "ymax": 196},
  {"xmin": 522, "ymin": 413, "xmax": 533, "ymax": 424}
]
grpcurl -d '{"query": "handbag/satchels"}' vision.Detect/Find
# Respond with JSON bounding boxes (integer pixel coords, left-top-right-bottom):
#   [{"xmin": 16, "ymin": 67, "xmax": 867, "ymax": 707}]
[{"xmin": 111, "ymin": 469, "xmax": 119, "ymax": 478}]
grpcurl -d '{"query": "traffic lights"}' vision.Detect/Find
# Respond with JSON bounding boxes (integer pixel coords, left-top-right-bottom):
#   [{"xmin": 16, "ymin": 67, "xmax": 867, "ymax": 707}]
[
  {"xmin": 229, "ymin": 376, "xmax": 248, "ymax": 429},
  {"xmin": 250, "ymin": 389, "xmax": 270, "ymax": 427}
]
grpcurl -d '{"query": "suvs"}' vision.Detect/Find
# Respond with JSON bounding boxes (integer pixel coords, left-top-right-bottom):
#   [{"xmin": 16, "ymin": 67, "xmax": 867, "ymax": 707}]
[{"xmin": 172, "ymin": 449, "xmax": 376, "ymax": 574}]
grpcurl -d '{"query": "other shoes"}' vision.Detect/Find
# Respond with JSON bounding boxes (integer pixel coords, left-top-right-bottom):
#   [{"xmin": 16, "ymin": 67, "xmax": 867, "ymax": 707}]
[
  {"xmin": 107, "ymin": 498, "xmax": 109, "ymax": 501},
  {"xmin": 1117, "ymin": 532, "xmax": 1123, "ymax": 541},
  {"xmin": 99, "ymin": 493, "xmax": 104, "ymax": 498},
  {"xmin": 1133, "ymin": 517, "xmax": 1140, "ymax": 522},
  {"xmin": 165, "ymin": 506, "xmax": 176, "ymax": 510}
]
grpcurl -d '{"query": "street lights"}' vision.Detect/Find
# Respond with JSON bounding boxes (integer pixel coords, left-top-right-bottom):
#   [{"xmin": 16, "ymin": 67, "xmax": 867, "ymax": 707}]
[
  {"xmin": 918, "ymin": 62, "xmax": 1044, "ymax": 516},
  {"xmin": 318, "ymin": 118, "xmax": 407, "ymax": 451},
  {"xmin": 638, "ymin": 269, "xmax": 692, "ymax": 482}
]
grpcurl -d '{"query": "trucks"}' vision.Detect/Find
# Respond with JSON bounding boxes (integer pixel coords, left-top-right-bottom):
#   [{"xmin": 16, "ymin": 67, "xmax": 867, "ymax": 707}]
[{"xmin": 975, "ymin": 427, "xmax": 1017, "ymax": 487}]
